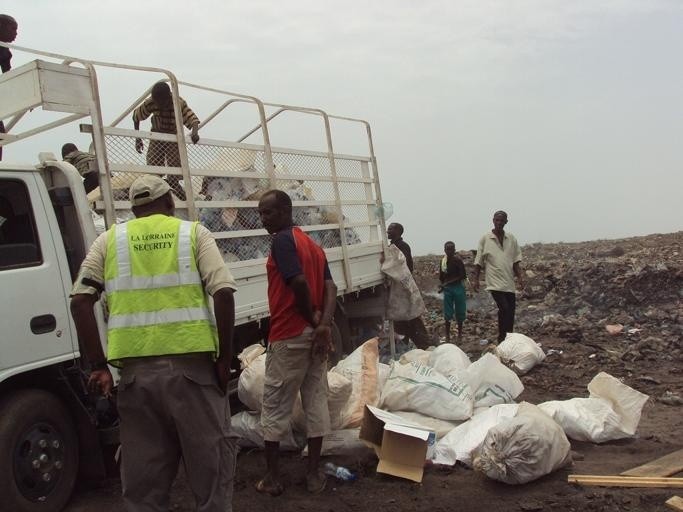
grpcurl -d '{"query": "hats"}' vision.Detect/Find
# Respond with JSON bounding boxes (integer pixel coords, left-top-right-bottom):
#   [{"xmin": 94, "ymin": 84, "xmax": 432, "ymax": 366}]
[{"xmin": 129, "ymin": 174, "xmax": 177, "ymax": 207}]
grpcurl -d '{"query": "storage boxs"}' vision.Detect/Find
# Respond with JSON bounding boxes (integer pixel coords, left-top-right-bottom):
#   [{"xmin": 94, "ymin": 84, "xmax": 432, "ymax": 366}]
[
  {"xmin": 376, "ymin": 424, "xmax": 430, "ymax": 485},
  {"xmin": 357, "ymin": 402, "xmax": 434, "ymax": 463}
]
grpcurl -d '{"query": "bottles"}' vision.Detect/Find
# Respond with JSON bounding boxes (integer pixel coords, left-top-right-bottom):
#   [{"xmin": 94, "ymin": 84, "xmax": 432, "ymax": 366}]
[{"xmin": 321, "ymin": 462, "xmax": 357, "ymax": 482}]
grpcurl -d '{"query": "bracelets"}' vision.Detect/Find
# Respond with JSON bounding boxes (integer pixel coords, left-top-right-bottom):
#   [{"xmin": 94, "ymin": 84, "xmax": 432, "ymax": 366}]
[{"xmin": 83, "ymin": 355, "xmax": 107, "ymax": 375}]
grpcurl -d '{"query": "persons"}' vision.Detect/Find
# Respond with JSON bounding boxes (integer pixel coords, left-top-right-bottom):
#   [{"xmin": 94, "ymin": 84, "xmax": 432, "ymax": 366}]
[
  {"xmin": 0, "ymin": 14, "xmax": 18, "ymax": 72},
  {"xmin": 132, "ymin": 80, "xmax": 201, "ymax": 200},
  {"xmin": 65, "ymin": 177, "xmax": 239, "ymax": 512},
  {"xmin": 437, "ymin": 240, "xmax": 468, "ymax": 342},
  {"xmin": 250, "ymin": 184, "xmax": 338, "ymax": 497},
  {"xmin": 61, "ymin": 143, "xmax": 98, "ymax": 194},
  {"xmin": 386, "ymin": 223, "xmax": 429, "ymax": 352},
  {"xmin": 473, "ymin": 210, "xmax": 525, "ymax": 340}
]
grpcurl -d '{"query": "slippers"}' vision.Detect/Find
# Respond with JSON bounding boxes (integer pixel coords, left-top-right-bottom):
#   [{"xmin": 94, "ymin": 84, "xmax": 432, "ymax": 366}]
[{"xmin": 255, "ymin": 471, "xmax": 329, "ymax": 497}]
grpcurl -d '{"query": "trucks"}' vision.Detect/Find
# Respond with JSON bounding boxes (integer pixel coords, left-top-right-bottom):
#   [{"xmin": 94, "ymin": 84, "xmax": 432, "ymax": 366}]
[{"xmin": 0, "ymin": 42, "xmax": 398, "ymax": 512}]
